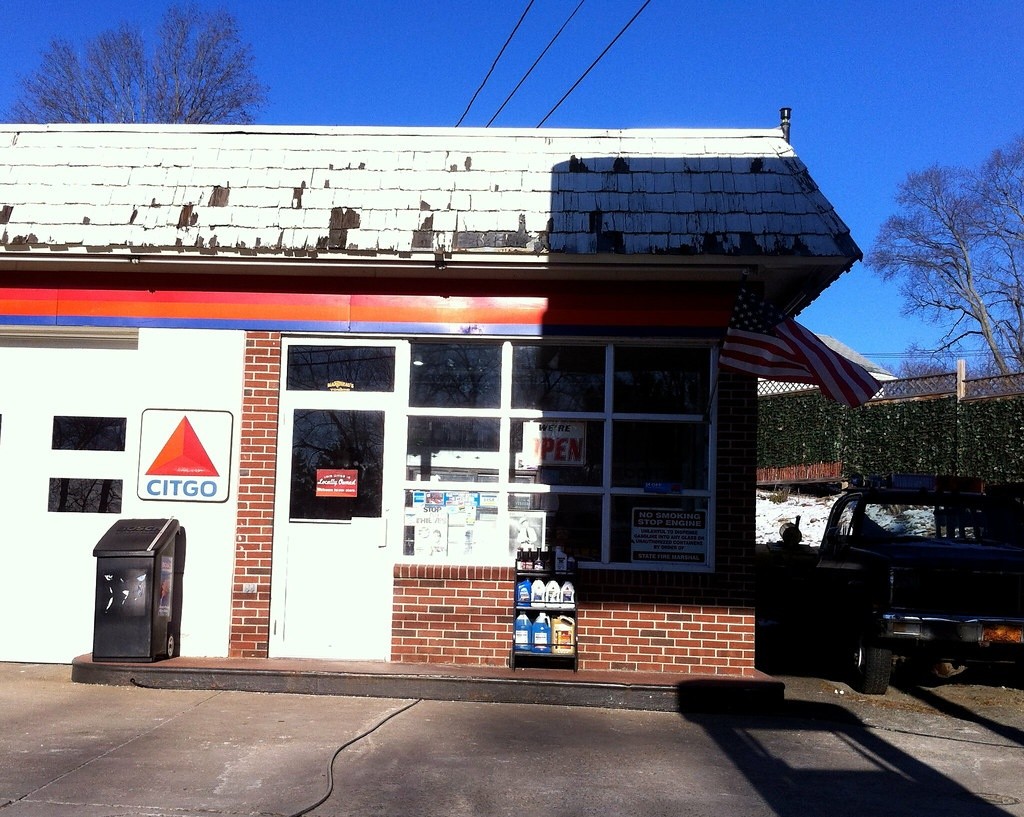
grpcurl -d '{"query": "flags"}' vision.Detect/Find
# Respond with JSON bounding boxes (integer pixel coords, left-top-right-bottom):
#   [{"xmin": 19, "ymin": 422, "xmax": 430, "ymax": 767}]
[{"xmin": 718, "ymin": 286, "xmax": 881, "ymax": 408}]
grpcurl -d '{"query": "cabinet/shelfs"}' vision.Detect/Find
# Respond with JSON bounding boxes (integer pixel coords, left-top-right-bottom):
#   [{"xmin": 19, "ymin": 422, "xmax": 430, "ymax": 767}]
[{"xmin": 510, "ymin": 552, "xmax": 579, "ymax": 673}]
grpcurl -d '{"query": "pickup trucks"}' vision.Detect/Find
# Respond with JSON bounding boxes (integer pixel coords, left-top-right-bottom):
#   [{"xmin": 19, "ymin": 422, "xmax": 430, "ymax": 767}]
[{"xmin": 817, "ymin": 475, "xmax": 1024, "ymax": 694}]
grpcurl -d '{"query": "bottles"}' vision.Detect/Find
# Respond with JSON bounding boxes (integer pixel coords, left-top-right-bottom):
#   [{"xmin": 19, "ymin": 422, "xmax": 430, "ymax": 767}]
[
  {"xmin": 516, "ymin": 546, "xmax": 575, "ymax": 574},
  {"xmin": 516, "ymin": 610, "xmax": 575, "ymax": 654},
  {"xmin": 516, "ymin": 577, "xmax": 575, "ymax": 608}
]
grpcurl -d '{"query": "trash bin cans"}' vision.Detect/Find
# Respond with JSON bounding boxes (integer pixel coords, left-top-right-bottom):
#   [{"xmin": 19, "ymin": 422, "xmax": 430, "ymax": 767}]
[{"xmin": 92, "ymin": 518, "xmax": 181, "ymax": 663}]
